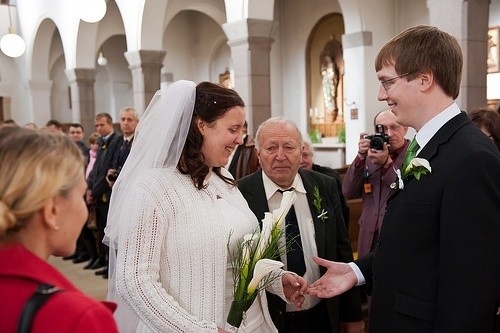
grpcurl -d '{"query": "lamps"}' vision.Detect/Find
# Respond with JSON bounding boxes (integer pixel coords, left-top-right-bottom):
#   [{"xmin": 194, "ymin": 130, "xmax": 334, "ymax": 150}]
[
  {"xmin": 97, "ymin": 52, "xmax": 108, "ymax": 67},
  {"xmin": 0, "ymin": 0, "xmax": 26, "ymax": 58}
]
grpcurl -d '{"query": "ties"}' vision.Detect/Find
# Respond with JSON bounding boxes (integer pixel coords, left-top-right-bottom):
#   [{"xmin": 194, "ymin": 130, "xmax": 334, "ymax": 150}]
[
  {"xmin": 277, "ymin": 189, "xmax": 307, "ymax": 278},
  {"xmin": 395, "ymin": 135, "xmax": 421, "ymax": 184}
]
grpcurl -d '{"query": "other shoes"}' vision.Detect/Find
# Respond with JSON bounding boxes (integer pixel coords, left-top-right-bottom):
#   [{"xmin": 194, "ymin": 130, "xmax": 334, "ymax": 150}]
[
  {"xmin": 84, "ymin": 258, "xmax": 97, "ymax": 270},
  {"xmin": 62, "ymin": 250, "xmax": 79, "ymax": 261},
  {"xmin": 72, "ymin": 252, "xmax": 90, "ymax": 264},
  {"xmin": 95, "ymin": 268, "xmax": 108, "ymax": 276},
  {"xmin": 89, "ymin": 259, "xmax": 107, "ymax": 270},
  {"xmin": 103, "ymin": 274, "xmax": 109, "ymax": 279}
]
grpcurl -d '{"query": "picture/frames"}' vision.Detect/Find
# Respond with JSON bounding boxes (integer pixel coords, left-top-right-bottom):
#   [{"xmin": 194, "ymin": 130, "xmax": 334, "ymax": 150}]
[{"xmin": 486, "ymin": 24, "xmax": 500, "ymax": 75}]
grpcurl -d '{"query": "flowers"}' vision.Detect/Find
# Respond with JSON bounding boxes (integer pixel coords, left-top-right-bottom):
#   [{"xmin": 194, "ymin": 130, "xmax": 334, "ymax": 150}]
[
  {"xmin": 224, "ymin": 173, "xmax": 309, "ymax": 333},
  {"xmin": 377, "ymin": 157, "xmax": 432, "ymax": 214}
]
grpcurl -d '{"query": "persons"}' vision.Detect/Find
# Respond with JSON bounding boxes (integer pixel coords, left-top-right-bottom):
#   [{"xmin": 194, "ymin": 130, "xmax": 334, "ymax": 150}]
[
  {"xmin": 343, "ymin": 109, "xmax": 412, "ymax": 260},
  {"xmin": 0, "ymin": 106, "xmax": 140, "ymax": 280},
  {"xmin": 234, "ymin": 117, "xmax": 364, "ymax": 333},
  {"xmin": 306, "ymin": 25, "xmax": 500, "ymax": 333},
  {"xmin": 223, "ymin": 120, "xmax": 355, "ymax": 262},
  {"xmin": 0, "ymin": 120, "xmax": 120, "ymax": 333},
  {"xmin": 102, "ymin": 79, "xmax": 311, "ymax": 333},
  {"xmin": 462, "ymin": 106, "xmax": 500, "ymax": 153}
]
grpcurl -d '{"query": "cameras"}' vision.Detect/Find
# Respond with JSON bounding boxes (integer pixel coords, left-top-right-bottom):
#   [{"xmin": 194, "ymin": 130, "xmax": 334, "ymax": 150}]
[{"xmin": 363, "ymin": 124, "xmax": 389, "ymax": 150}]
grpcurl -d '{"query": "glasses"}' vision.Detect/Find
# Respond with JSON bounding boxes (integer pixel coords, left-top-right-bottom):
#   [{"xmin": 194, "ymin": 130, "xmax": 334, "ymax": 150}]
[{"xmin": 381, "ymin": 68, "xmax": 422, "ymax": 90}]
